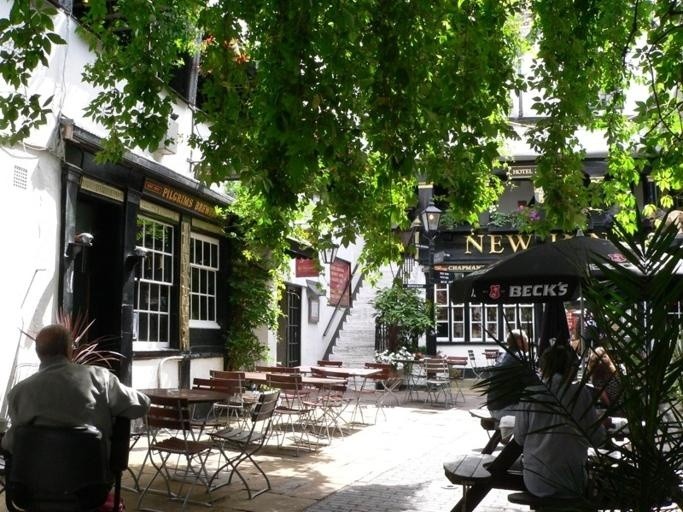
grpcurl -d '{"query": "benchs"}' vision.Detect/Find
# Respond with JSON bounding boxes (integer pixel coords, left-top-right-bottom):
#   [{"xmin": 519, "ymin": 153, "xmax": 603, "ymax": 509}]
[{"xmin": 442, "ymin": 407, "xmax": 647, "ymax": 512}]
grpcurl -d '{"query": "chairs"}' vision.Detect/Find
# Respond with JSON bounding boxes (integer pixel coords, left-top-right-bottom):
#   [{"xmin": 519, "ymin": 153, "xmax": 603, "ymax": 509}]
[
  {"xmin": 5, "ymin": 416, "xmax": 130, "ymax": 512},
  {"xmin": 468, "ymin": 350, "xmax": 484, "ymax": 384},
  {"xmin": 136, "ymin": 349, "xmax": 467, "ymax": 512}
]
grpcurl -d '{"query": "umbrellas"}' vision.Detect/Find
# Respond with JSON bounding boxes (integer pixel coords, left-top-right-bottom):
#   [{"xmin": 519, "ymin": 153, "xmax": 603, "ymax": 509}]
[
  {"xmin": 450, "ymin": 230, "xmax": 683, "ymax": 383},
  {"xmin": 537, "ymin": 301, "xmax": 570, "ymax": 362}
]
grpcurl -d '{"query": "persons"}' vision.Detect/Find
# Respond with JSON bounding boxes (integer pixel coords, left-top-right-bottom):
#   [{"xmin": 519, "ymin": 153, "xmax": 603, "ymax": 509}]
[
  {"xmin": 2, "ymin": 324, "xmax": 151, "ymax": 458},
  {"xmin": 587, "ymin": 346, "xmax": 627, "ymax": 428},
  {"xmin": 515, "ymin": 343, "xmax": 607, "ymax": 498},
  {"xmin": 487, "ymin": 329, "xmax": 543, "ymax": 420}
]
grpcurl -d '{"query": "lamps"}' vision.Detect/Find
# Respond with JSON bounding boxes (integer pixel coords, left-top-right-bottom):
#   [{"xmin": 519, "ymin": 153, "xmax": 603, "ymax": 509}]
[
  {"xmin": 124, "ymin": 247, "xmax": 147, "ymax": 277},
  {"xmin": 64, "ymin": 233, "xmax": 94, "ymax": 271}
]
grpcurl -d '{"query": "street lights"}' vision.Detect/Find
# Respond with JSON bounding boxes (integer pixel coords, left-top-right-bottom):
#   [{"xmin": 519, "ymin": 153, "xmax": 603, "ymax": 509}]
[{"xmin": 420, "ymin": 196, "xmax": 442, "ymax": 392}]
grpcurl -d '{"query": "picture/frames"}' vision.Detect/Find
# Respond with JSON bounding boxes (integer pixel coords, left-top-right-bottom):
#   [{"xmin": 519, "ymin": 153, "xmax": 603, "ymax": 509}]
[
  {"xmin": 326, "ymin": 256, "xmax": 353, "ymax": 308},
  {"xmin": 308, "ymin": 298, "xmax": 320, "ymax": 322},
  {"xmin": 296, "ymin": 257, "xmax": 319, "ymax": 277}
]
